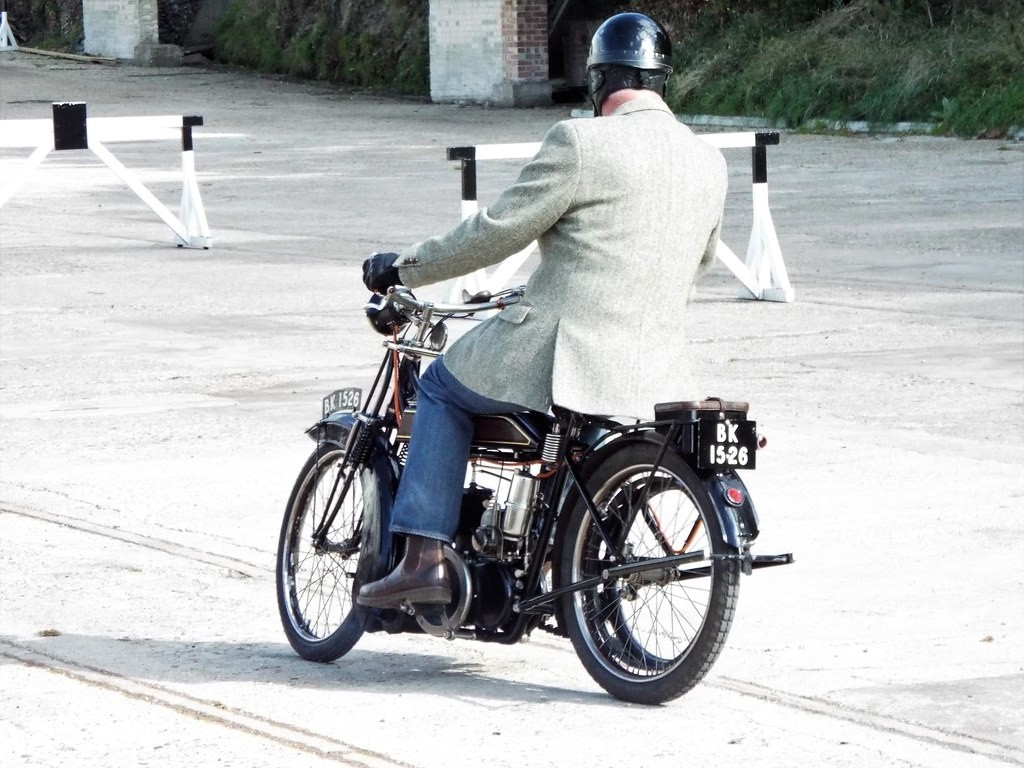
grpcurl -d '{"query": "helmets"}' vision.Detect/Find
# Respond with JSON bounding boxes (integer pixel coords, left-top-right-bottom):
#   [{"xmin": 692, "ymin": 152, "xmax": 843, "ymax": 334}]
[{"xmin": 585, "ymin": 12, "xmax": 673, "ymax": 75}]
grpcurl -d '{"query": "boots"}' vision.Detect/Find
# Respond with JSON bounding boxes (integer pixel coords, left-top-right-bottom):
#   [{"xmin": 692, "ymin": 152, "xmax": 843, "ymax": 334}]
[
  {"xmin": 355, "ymin": 533, "xmax": 453, "ymax": 607},
  {"xmin": 530, "ymin": 542, "xmax": 554, "ymax": 575}
]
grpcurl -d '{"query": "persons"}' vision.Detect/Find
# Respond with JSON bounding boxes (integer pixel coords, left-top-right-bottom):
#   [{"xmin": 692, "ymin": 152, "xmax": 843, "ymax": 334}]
[{"xmin": 355, "ymin": 11, "xmax": 729, "ymax": 607}]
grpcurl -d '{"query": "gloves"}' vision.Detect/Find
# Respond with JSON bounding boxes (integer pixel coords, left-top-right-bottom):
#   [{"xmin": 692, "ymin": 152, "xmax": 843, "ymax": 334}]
[{"xmin": 363, "ymin": 252, "xmax": 402, "ymax": 294}]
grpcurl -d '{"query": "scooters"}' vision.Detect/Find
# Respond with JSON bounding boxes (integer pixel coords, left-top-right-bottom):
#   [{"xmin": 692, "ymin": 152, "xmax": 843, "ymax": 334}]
[{"xmin": 274, "ymin": 260, "xmax": 795, "ymax": 708}]
